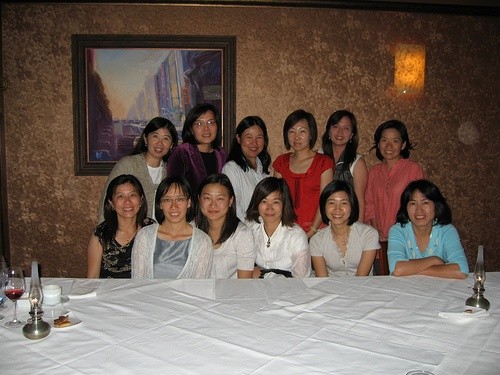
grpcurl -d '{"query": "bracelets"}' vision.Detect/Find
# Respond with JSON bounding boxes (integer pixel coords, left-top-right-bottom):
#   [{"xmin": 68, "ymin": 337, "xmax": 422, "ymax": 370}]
[{"xmin": 309, "ymin": 226, "xmax": 318, "ymax": 232}]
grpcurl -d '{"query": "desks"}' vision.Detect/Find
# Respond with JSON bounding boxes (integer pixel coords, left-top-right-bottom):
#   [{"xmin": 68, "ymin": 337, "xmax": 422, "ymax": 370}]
[{"xmin": 0, "ymin": 271, "xmax": 500, "ymax": 375}]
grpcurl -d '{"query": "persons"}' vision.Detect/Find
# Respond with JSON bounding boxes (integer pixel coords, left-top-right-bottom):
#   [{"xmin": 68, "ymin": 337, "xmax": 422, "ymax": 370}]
[
  {"xmin": 243, "ymin": 177, "xmax": 312, "ymax": 278},
  {"xmin": 315, "ymin": 110, "xmax": 368, "ymax": 224},
  {"xmin": 86, "ymin": 174, "xmax": 157, "ymax": 280},
  {"xmin": 272, "ymin": 109, "xmax": 335, "ymax": 240},
  {"xmin": 310, "ymin": 180, "xmax": 381, "ymax": 277},
  {"xmin": 189, "ymin": 173, "xmax": 256, "ymax": 280},
  {"xmin": 131, "ymin": 176, "xmax": 213, "ymax": 280},
  {"xmin": 388, "ymin": 179, "xmax": 470, "ymax": 279},
  {"xmin": 99, "ymin": 117, "xmax": 178, "ymax": 223},
  {"xmin": 365, "ymin": 120, "xmax": 426, "ymax": 276},
  {"xmin": 167, "ymin": 103, "xmax": 229, "ymax": 222},
  {"xmin": 222, "ymin": 116, "xmax": 272, "ymax": 223}
]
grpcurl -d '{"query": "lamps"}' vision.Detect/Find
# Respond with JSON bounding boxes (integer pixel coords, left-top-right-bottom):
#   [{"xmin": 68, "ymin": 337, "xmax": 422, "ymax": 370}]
[
  {"xmin": 466, "ymin": 246, "xmax": 490, "ymax": 311},
  {"xmin": 22, "ymin": 260, "xmax": 51, "ymax": 340}
]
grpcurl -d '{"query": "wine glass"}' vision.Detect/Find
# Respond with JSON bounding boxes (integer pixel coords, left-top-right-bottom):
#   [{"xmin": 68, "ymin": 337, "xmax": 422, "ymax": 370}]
[{"xmin": 0, "ymin": 262, "xmax": 25, "ymax": 327}]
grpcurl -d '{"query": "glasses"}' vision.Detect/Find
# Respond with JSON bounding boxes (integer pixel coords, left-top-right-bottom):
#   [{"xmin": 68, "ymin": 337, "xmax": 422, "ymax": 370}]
[
  {"xmin": 162, "ymin": 195, "xmax": 190, "ymax": 203},
  {"xmin": 193, "ymin": 120, "xmax": 216, "ymax": 127}
]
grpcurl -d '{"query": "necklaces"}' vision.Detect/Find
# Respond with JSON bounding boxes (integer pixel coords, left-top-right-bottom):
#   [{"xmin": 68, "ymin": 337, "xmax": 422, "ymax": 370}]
[
  {"xmin": 331, "ymin": 221, "xmax": 349, "ymax": 246},
  {"xmin": 143, "ymin": 157, "xmax": 161, "ymax": 168},
  {"xmin": 263, "ymin": 218, "xmax": 283, "ymax": 248},
  {"xmin": 116, "ymin": 225, "xmax": 139, "ymax": 244}
]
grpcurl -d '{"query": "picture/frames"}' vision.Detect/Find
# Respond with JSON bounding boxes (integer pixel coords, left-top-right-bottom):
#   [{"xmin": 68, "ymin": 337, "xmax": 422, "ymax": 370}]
[{"xmin": 71, "ymin": 35, "xmax": 237, "ymax": 176}]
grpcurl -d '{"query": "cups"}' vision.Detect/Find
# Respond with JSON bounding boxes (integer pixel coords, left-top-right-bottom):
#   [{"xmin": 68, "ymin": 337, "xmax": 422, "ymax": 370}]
[{"xmin": 42, "ymin": 285, "xmax": 63, "ymax": 305}]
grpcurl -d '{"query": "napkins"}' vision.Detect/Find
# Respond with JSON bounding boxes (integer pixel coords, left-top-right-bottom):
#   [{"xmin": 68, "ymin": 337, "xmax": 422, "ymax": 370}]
[
  {"xmin": 68, "ymin": 278, "xmax": 101, "ymax": 300},
  {"xmin": 439, "ymin": 305, "xmax": 489, "ymax": 324}
]
